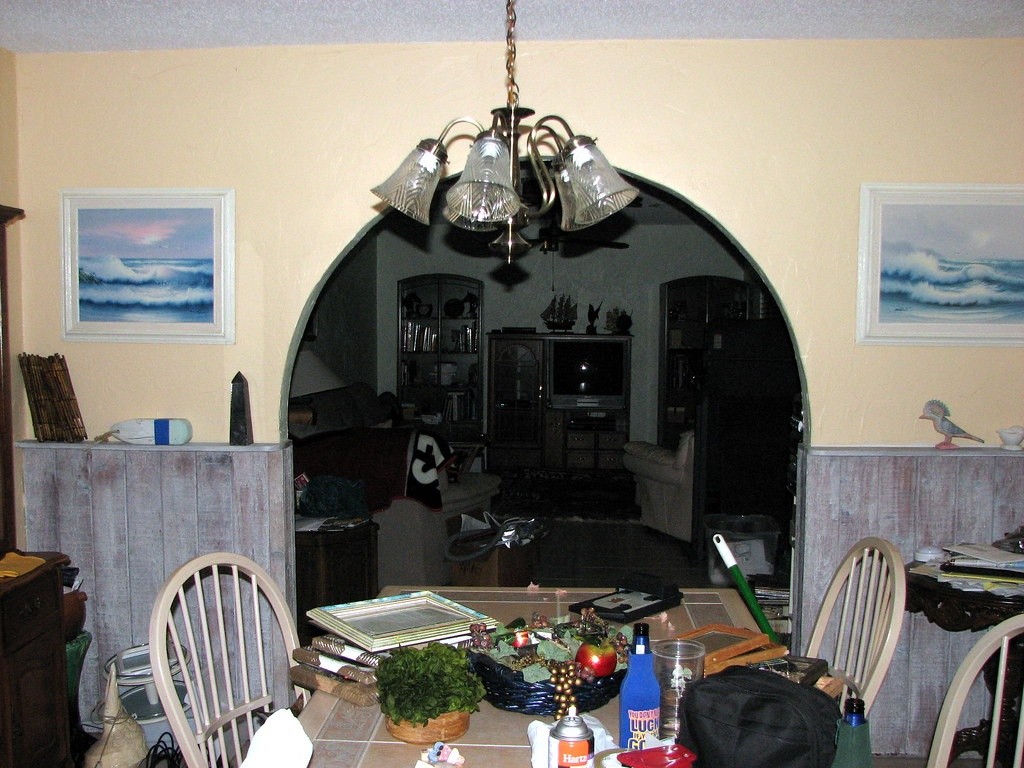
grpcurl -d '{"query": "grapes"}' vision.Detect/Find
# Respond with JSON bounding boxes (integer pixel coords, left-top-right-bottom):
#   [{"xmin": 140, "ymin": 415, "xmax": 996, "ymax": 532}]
[
  {"xmin": 532, "ymin": 606, "xmax": 630, "ymax": 721},
  {"xmin": 468, "ymin": 622, "xmax": 494, "ymax": 649}
]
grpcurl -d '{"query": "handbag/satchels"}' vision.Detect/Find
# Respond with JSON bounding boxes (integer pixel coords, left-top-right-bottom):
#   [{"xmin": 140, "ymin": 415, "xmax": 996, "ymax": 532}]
[
  {"xmin": 444, "ymin": 510, "xmax": 553, "ymax": 563},
  {"xmin": 674, "ymin": 663, "xmax": 841, "ymax": 768}
]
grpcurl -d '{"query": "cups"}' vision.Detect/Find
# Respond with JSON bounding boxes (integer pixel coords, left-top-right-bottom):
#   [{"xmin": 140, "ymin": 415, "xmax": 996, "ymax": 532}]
[
  {"xmin": 652, "ymin": 638, "xmax": 705, "ymax": 739},
  {"xmin": 446, "ymin": 464, "xmax": 463, "ymax": 482}
]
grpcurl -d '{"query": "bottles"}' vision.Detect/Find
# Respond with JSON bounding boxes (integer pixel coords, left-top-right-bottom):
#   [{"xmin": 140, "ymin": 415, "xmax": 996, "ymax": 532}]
[
  {"xmin": 843, "ymin": 698, "xmax": 867, "ymax": 727},
  {"xmin": 548, "ymin": 706, "xmax": 594, "ymax": 768},
  {"xmin": 619, "ymin": 623, "xmax": 660, "ymax": 749},
  {"xmin": 464, "ymin": 389, "xmax": 475, "ymax": 421}
]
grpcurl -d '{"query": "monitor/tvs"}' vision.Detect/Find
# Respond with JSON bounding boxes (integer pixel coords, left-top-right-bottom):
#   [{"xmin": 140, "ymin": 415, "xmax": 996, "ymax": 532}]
[{"xmin": 546, "ymin": 334, "xmax": 631, "ymax": 409}]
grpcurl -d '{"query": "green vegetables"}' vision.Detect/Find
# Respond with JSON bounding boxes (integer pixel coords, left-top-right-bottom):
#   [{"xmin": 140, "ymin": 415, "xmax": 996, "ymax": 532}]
[{"xmin": 375, "ymin": 640, "xmax": 486, "ymax": 728}]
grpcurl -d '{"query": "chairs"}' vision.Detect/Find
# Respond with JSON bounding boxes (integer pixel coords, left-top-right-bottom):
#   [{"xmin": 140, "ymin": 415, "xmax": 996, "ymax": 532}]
[
  {"xmin": 806, "ymin": 537, "xmax": 905, "ymax": 720},
  {"xmin": 927, "ymin": 613, "xmax": 1024, "ymax": 768},
  {"xmin": 148, "ymin": 554, "xmax": 312, "ymax": 768}
]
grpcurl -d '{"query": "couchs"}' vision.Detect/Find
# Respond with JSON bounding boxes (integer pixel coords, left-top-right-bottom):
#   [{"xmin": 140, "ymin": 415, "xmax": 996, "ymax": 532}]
[
  {"xmin": 288, "ymin": 382, "xmax": 501, "ymax": 590},
  {"xmin": 623, "ymin": 429, "xmax": 696, "ymax": 543}
]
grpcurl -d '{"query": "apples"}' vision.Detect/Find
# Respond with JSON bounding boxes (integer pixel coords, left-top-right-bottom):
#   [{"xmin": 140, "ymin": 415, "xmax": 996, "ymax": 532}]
[{"xmin": 576, "ymin": 640, "xmax": 618, "ymax": 677}]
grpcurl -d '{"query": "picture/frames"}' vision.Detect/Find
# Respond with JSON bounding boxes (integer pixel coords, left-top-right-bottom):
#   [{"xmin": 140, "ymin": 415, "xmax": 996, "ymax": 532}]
[
  {"xmin": 657, "ymin": 624, "xmax": 844, "ymax": 698},
  {"xmin": 59, "ymin": 187, "xmax": 236, "ymax": 344},
  {"xmin": 856, "ymin": 182, "xmax": 1024, "ymax": 347},
  {"xmin": 289, "ymin": 590, "xmax": 497, "ymax": 706}
]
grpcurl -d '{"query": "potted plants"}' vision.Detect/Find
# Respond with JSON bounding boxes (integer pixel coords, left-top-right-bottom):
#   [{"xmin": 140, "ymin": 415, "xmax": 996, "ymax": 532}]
[{"xmin": 375, "ymin": 642, "xmax": 487, "ymax": 744}]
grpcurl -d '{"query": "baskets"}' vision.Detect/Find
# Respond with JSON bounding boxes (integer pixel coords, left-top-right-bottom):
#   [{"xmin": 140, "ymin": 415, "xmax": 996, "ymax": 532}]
[
  {"xmin": 464, "ymin": 643, "xmax": 632, "ymax": 716},
  {"xmin": 385, "ymin": 708, "xmax": 470, "ymax": 745}
]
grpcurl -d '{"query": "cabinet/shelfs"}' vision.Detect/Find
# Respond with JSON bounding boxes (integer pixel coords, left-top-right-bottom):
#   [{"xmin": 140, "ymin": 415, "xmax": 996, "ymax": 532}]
[
  {"xmin": 395, "ymin": 273, "xmax": 483, "ymax": 436},
  {"xmin": 658, "ymin": 275, "xmax": 800, "ymax": 570},
  {"xmin": 486, "ymin": 332, "xmax": 630, "ymax": 468},
  {"xmin": 0, "ymin": 205, "xmax": 75, "ymax": 768}
]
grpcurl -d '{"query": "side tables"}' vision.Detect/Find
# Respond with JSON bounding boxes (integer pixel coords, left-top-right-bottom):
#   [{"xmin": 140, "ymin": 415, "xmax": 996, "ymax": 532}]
[{"xmin": 295, "ymin": 521, "xmax": 379, "ymax": 649}]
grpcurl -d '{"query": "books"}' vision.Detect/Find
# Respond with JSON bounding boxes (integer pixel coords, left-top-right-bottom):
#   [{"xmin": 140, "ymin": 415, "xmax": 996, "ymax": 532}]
[
  {"xmin": 458, "ymin": 322, "xmax": 479, "ymax": 354},
  {"xmin": 402, "ymin": 318, "xmax": 438, "ymax": 352},
  {"xmin": 400, "ymin": 358, "xmax": 417, "ymax": 385},
  {"xmin": 443, "ymin": 390, "xmax": 479, "ymax": 423}
]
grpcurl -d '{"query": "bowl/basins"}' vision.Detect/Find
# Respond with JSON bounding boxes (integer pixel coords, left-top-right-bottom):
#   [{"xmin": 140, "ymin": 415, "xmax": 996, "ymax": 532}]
[
  {"xmin": 995, "ymin": 425, "xmax": 1024, "ymax": 450},
  {"xmin": 468, "ymin": 647, "xmax": 628, "ymax": 716}
]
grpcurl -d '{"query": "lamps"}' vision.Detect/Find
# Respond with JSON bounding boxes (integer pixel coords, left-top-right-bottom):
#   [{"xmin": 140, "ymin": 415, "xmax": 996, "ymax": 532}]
[
  {"xmin": 288, "ymin": 351, "xmax": 348, "ymax": 424},
  {"xmin": 370, "ymin": 0, "xmax": 640, "ymax": 264}
]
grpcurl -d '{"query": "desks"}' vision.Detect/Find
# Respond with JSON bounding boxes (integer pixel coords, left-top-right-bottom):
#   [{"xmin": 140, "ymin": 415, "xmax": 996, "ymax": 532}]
[
  {"xmin": 905, "ymin": 561, "xmax": 1024, "ymax": 768},
  {"xmin": 292, "ymin": 587, "xmax": 761, "ymax": 767}
]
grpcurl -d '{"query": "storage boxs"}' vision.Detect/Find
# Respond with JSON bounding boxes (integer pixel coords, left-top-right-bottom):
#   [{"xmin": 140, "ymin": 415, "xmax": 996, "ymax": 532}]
[
  {"xmin": 452, "ymin": 543, "xmax": 525, "ymax": 587},
  {"xmin": 704, "ymin": 514, "xmax": 780, "ymax": 586}
]
